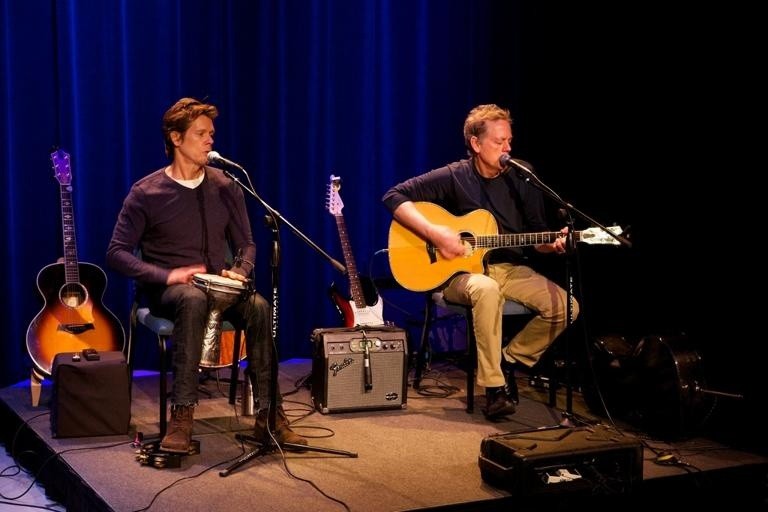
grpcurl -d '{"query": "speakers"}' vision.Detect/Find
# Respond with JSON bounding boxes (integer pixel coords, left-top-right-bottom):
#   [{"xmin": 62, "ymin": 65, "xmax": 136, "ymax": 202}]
[{"xmin": 310, "ymin": 325, "xmax": 409, "ymax": 417}]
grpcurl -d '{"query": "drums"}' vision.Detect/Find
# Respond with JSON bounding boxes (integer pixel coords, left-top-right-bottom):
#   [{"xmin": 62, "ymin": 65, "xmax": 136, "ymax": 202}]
[{"xmin": 191, "ymin": 273, "xmax": 246, "ymax": 367}]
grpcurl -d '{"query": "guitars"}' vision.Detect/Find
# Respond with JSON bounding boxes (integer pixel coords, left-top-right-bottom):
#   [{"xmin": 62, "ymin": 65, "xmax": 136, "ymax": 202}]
[
  {"xmin": 26, "ymin": 150, "xmax": 125, "ymax": 377},
  {"xmin": 387, "ymin": 201, "xmax": 624, "ymax": 293},
  {"xmin": 325, "ymin": 174, "xmax": 384, "ymax": 328}
]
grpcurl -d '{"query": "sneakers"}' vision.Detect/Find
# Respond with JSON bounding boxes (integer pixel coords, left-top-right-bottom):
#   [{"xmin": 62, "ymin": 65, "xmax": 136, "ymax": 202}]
[
  {"xmin": 501, "ymin": 349, "xmax": 520, "ymax": 404},
  {"xmin": 486, "ymin": 386, "xmax": 515, "ymax": 419}
]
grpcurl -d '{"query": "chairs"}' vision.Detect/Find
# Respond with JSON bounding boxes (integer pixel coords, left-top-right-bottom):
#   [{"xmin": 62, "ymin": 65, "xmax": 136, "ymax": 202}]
[
  {"xmin": 126, "ymin": 250, "xmax": 245, "ymax": 439},
  {"xmin": 413, "ymin": 290, "xmax": 557, "ymax": 414}
]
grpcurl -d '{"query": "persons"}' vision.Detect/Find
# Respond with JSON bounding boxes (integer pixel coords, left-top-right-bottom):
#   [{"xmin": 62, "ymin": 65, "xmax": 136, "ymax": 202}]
[
  {"xmin": 106, "ymin": 97, "xmax": 308, "ymax": 453},
  {"xmin": 379, "ymin": 103, "xmax": 580, "ymax": 418}
]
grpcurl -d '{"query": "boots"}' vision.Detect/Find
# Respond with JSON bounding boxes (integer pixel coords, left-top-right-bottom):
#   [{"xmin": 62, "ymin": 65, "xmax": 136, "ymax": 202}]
[
  {"xmin": 254, "ymin": 406, "xmax": 307, "ymax": 452},
  {"xmin": 159, "ymin": 403, "xmax": 194, "ymax": 454}
]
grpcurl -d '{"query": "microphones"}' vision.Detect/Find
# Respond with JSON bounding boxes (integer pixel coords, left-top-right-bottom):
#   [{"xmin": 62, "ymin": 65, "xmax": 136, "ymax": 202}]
[
  {"xmin": 206, "ymin": 150, "xmax": 243, "ymax": 172},
  {"xmin": 362, "ymin": 343, "xmax": 373, "ymax": 390},
  {"xmin": 499, "ymin": 153, "xmax": 532, "ymax": 176}
]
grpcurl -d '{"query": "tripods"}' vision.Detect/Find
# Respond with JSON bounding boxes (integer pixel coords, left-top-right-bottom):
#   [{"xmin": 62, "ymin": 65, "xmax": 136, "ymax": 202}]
[
  {"xmin": 219, "ymin": 170, "xmax": 359, "ymax": 476},
  {"xmin": 488, "ymin": 180, "xmax": 632, "ymax": 437}
]
